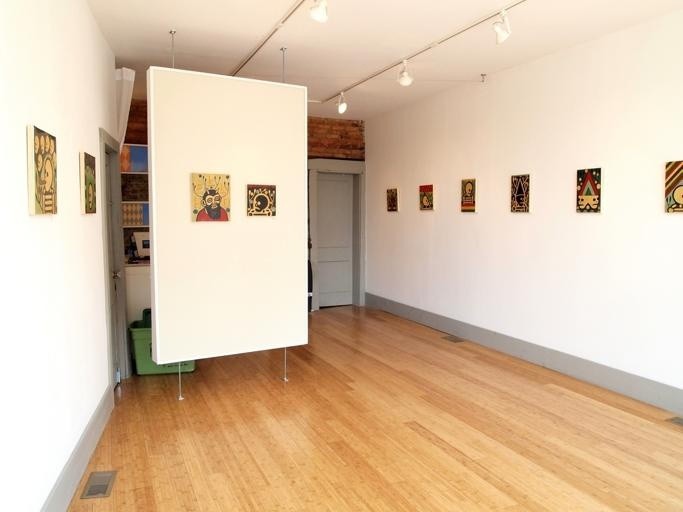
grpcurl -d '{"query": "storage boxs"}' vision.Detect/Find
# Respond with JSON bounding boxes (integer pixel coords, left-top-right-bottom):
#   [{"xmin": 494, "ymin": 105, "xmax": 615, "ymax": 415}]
[{"xmin": 128, "ymin": 320, "xmax": 197, "ymax": 377}]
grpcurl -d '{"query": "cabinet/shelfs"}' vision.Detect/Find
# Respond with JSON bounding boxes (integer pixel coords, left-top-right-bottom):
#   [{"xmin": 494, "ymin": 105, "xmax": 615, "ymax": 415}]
[
  {"xmin": 117, "ymin": 143, "xmax": 150, "ymax": 229},
  {"xmin": 123, "ymin": 258, "xmax": 151, "ymax": 326}
]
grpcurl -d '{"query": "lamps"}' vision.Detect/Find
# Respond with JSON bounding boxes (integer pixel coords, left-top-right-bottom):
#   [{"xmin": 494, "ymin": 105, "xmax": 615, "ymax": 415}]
[
  {"xmin": 491, "ymin": 5, "xmax": 512, "ymax": 45},
  {"xmin": 308, "ymin": 0, "xmax": 328, "ymax": 23},
  {"xmin": 396, "ymin": 56, "xmax": 416, "ymax": 89},
  {"xmin": 334, "ymin": 89, "xmax": 349, "ymax": 114}
]
